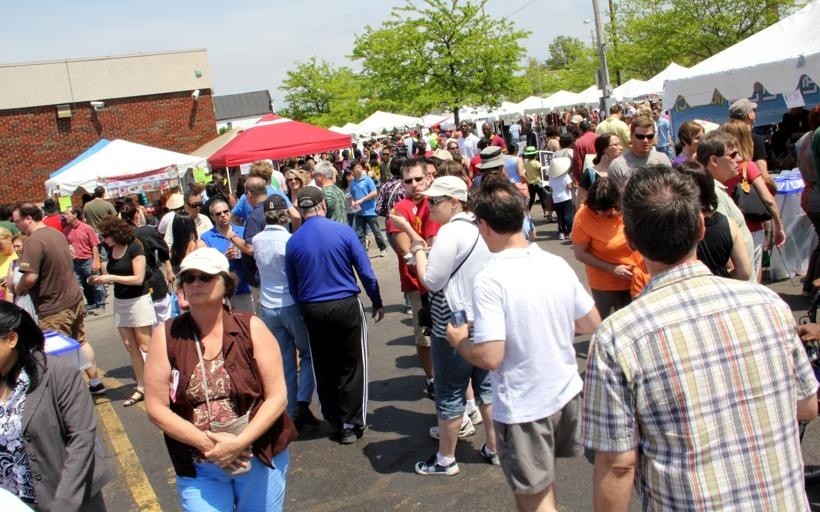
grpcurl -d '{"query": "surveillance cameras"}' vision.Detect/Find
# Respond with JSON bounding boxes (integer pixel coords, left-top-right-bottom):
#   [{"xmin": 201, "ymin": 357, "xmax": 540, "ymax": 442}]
[
  {"xmin": 90, "ymin": 101, "xmax": 105, "ymax": 110},
  {"xmin": 191, "ymin": 89, "xmax": 201, "ymax": 101}
]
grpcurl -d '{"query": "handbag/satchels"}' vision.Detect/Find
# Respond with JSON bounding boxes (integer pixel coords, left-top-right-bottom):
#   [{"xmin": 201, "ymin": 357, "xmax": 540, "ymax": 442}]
[
  {"xmin": 735, "ymin": 180, "xmax": 772, "ymax": 222},
  {"xmin": 146, "ymin": 271, "xmax": 167, "ymax": 301},
  {"xmin": 208, "ymin": 415, "xmax": 253, "ymax": 477},
  {"xmin": 417, "ymin": 308, "xmax": 433, "ymax": 336}
]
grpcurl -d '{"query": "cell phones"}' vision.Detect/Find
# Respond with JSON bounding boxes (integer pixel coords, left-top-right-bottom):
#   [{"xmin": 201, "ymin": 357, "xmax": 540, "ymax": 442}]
[{"xmin": 451, "ymin": 309, "xmax": 471, "ymax": 338}]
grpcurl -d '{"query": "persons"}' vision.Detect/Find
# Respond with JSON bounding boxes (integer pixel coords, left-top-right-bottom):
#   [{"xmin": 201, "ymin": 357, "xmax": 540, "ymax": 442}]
[
  {"xmin": 86, "ymin": 217, "xmax": 158, "ymax": 408},
  {"xmin": 274, "ymin": 98, "xmax": 819, "ymax": 372},
  {"xmin": 0, "ymin": 301, "xmax": 112, "ymax": 512},
  {"xmin": 410, "ymin": 175, "xmax": 502, "ymax": 475},
  {"xmin": 574, "ymin": 162, "xmax": 819, "ymax": 512},
  {"xmin": 386, "ymin": 158, "xmax": 443, "ymax": 400},
  {"xmin": 0, "ymin": 160, "xmax": 273, "ymax": 316},
  {"xmin": 446, "ymin": 183, "xmax": 601, "ymax": 512},
  {"xmin": 388, "ymin": 212, "xmax": 483, "ymax": 440},
  {"xmin": 252, "ymin": 193, "xmax": 321, "ymax": 440},
  {"xmin": 12, "ymin": 202, "xmax": 106, "ymax": 395},
  {"xmin": 285, "ymin": 186, "xmax": 385, "ymax": 444},
  {"xmin": 143, "ymin": 247, "xmax": 299, "ymax": 512}
]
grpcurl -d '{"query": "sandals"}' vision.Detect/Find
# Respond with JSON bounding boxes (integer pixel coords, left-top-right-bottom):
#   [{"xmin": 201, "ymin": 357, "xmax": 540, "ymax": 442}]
[{"xmin": 123, "ymin": 389, "xmax": 144, "ymax": 407}]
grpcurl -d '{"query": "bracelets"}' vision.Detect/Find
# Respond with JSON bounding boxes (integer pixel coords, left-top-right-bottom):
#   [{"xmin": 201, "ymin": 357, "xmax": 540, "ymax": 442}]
[{"xmin": 414, "ymin": 248, "xmax": 425, "ymax": 256}]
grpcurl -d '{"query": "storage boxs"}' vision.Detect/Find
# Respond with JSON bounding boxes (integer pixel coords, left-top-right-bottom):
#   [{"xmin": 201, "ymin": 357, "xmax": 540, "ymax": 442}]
[
  {"xmin": 44, "ymin": 332, "xmax": 92, "ymax": 375},
  {"xmin": 768, "ymin": 173, "xmax": 807, "ymax": 278}
]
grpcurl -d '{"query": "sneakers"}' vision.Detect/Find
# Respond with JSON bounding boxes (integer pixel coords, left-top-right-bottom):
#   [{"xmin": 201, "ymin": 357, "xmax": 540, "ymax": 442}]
[
  {"xmin": 88, "ymin": 308, "xmax": 104, "ymax": 314},
  {"xmin": 88, "ymin": 383, "xmax": 106, "ymax": 394},
  {"xmin": 292, "ymin": 381, "xmax": 501, "ymax": 475}
]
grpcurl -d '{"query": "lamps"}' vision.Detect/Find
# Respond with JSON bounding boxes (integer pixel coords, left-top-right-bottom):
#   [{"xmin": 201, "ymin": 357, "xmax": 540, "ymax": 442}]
[{"xmin": 57, "ymin": 105, "xmax": 72, "ymax": 118}]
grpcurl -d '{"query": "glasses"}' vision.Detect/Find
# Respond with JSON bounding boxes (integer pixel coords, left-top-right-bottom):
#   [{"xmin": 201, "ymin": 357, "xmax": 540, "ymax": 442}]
[
  {"xmin": 724, "ymin": 151, "xmax": 740, "ymax": 159},
  {"xmin": 181, "ymin": 274, "xmax": 213, "ymax": 283},
  {"xmin": 402, "ymin": 176, "xmax": 424, "ymax": 184},
  {"xmin": 214, "ymin": 210, "xmax": 229, "ymax": 216},
  {"xmin": 287, "ymin": 177, "xmax": 299, "ymax": 182},
  {"xmin": 187, "ymin": 201, "xmax": 203, "ymax": 208},
  {"xmin": 633, "ymin": 131, "xmax": 655, "ymax": 140}
]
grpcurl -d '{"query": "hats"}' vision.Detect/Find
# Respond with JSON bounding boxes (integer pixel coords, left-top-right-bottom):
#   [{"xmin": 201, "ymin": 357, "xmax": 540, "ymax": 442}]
[
  {"xmin": 730, "ymin": 98, "xmax": 758, "ymax": 119},
  {"xmin": 296, "ymin": 186, "xmax": 323, "ymax": 208},
  {"xmin": 176, "ymin": 247, "xmax": 230, "ymax": 277},
  {"xmin": 166, "ymin": 193, "xmax": 184, "ymax": 209},
  {"xmin": 263, "ymin": 195, "xmax": 287, "ymax": 211},
  {"xmin": 419, "ymin": 114, "xmax": 585, "ymax": 204}
]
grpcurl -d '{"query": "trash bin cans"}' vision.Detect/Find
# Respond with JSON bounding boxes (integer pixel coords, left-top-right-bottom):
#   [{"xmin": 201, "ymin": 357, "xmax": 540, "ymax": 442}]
[{"xmin": 766, "ymin": 175, "xmax": 819, "ymax": 282}]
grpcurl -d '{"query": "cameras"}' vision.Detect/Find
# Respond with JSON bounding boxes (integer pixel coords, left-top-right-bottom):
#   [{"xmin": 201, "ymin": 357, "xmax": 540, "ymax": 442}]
[{"xmin": 417, "ymin": 308, "xmax": 431, "ymax": 327}]
